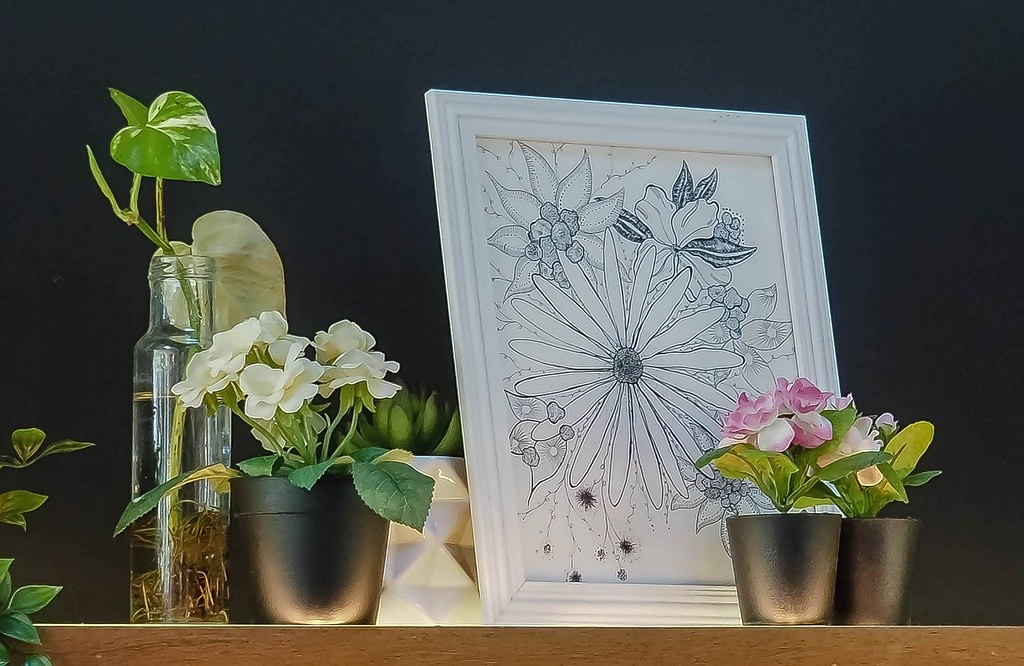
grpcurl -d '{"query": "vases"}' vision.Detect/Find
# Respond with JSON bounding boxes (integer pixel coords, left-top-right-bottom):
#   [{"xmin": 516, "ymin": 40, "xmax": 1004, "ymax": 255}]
[
  {"xmin": 831, "ymin": 517, "xmax": 923, "ymax": 626},
  {"xmin": 218, "ymin": 471, "xmax": 391, "ymax": 627},
  {"xmin": 723, "ymin": 513, "xmax": 842, "ymax": 626},
  {"xmin": 131, "ymin": 255, "xmax": 231, "ymax": 626}
]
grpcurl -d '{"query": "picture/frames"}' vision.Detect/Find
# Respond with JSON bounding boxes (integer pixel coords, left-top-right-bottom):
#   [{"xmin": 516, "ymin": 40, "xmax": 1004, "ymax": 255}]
[{"xmin": 423, "ymin": 85, "xmax": 843, "ymax": 626}]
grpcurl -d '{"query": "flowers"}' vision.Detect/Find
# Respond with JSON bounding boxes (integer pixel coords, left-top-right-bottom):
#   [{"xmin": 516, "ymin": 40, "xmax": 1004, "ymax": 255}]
[
  {"xmin": 827, "ymin": 391, "xmax": 944, "ymax": 517},
  {"xmin": 110, "ymin": 308, "xmax": 440, "ymax": 537},
  {"xmin": 691, "ymin": 375, "xmax": 895, "ymax": 512}
]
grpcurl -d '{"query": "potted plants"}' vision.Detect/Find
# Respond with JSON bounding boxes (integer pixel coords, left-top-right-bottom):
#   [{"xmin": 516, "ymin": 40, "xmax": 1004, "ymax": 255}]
[{"xmin": 342, "ymin": 378, "xmax": 483, "ymax": 629}]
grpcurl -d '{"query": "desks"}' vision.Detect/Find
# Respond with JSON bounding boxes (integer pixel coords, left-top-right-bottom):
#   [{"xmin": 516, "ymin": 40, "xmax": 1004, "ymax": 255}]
[{"xmin": 27, "ymin": 624, "xmax": 1024, "ymax": 666}]
